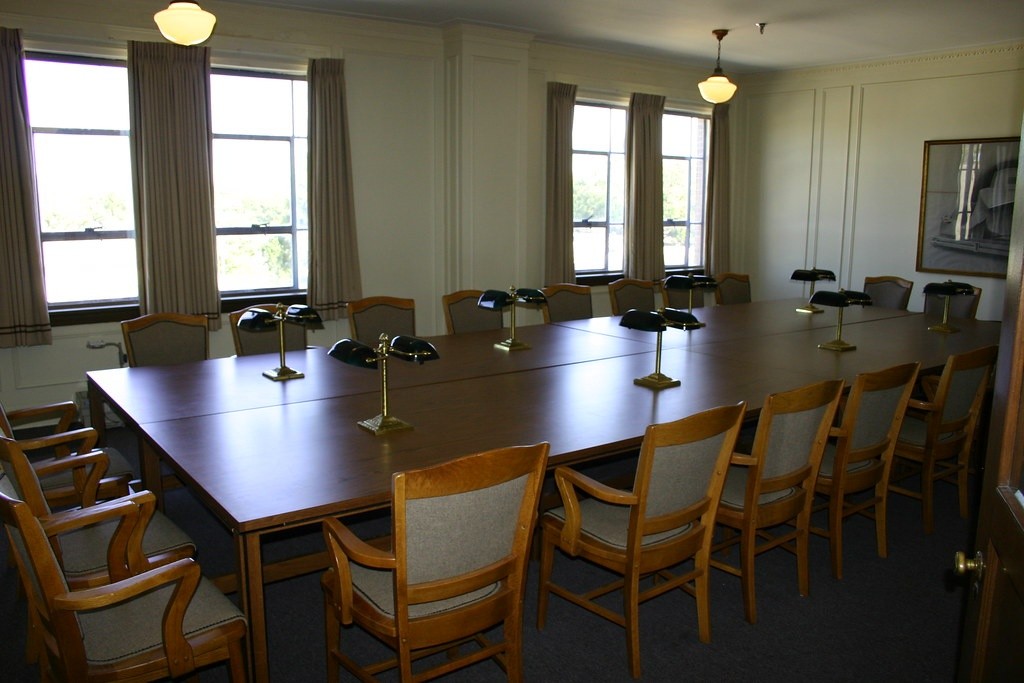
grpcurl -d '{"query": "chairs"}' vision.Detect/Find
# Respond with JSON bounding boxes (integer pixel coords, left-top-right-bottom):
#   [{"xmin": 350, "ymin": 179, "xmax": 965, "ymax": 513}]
[
  {"xmin": 0, "ymin": 400, "xmax": 248, "ymax": 683},
  {"xmin": 121, "ymin": 274, "xmax": 1000, "ymax": 683}
]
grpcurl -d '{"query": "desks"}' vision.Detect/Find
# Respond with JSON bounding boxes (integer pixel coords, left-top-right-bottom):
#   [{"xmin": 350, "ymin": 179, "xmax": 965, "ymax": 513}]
[{"xmin": 87, "ymin": 295, "xmax": 1000, "ymax": 683}]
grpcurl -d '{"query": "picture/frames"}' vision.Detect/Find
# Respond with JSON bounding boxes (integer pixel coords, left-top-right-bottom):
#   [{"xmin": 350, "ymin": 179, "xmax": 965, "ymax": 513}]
[{"xmin": 916, "ymin": 137, "xmax": 1021, "ymax": 279}]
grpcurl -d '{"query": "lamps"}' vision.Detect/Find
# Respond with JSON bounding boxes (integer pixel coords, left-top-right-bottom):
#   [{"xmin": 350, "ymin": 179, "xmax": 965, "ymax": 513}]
[
  {"xmin": 924, "ymin": 279, "xmax": 974, "ymax": 334},
  {"xmin": 697, "ymin": 30, "xmax": 738, "ymax": 104},
  {"xmin": 477, "ymin": 285, "xmax": 548, "ymax": 352},
  {"xmin": 808, "ymin": 288, "xmax": 873, "ymax": 351},
  {"xmin": 620, "ymin": 306, "xmax": 706, "ymax": 389},
  {"xmin": 154, "ymin": 0, "xmax": 217, "ymax": 47},
  {"xmin": 791, "ymin": 267, "xmax": 836, "ymax": 313},
  {"xmin": 236, "ymin": 302, "xmax": 323, "ymax": 381},
  {"xmin": 327, "ymin": 332, "xmax": 441, "ymax": 437},
  {"xmin": 663, "ymin": 273, "xmax": 719, "ymax": 314}
]
